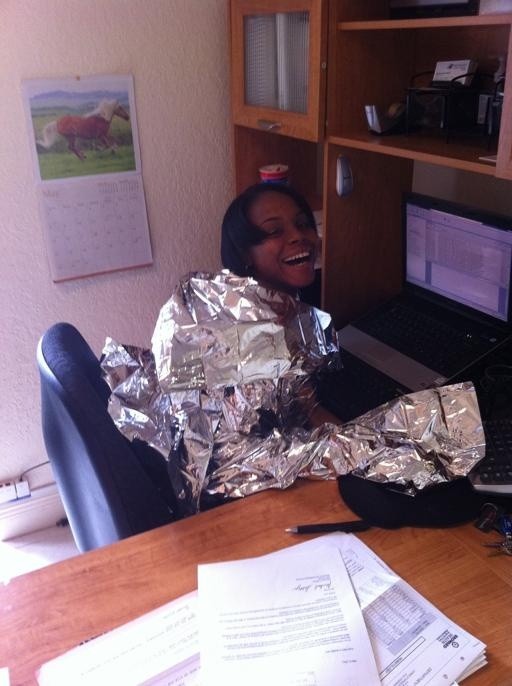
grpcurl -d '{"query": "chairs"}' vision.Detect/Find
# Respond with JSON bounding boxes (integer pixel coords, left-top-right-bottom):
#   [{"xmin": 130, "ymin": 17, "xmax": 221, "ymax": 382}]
[{"xmin": 37, "ymin": 323, "xmax": 174, "ymax": 554}]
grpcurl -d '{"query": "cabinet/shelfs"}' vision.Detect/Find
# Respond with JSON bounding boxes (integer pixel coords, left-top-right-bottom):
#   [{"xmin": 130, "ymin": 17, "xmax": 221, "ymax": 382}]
[{"xmin": 226, "ymin": 0, "xmax": 512, "ymax": 404}]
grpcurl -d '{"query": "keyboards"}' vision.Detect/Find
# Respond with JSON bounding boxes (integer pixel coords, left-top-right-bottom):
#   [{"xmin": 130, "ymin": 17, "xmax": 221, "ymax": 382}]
[{"xmin": 465, "ymin": 415, "xmax": 512, "ymax": 496}]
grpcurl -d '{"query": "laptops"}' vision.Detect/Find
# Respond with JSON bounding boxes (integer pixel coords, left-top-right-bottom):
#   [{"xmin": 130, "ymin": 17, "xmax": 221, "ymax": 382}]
[{"xmin": 334, "ymin": 190, "xmax": 512, "ymax": 393}]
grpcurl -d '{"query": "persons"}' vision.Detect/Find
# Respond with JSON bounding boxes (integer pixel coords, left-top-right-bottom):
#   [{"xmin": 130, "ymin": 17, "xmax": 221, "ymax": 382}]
[{"xmin": 155, "ymin": 181, "xmax": 485, "ymax": 483}]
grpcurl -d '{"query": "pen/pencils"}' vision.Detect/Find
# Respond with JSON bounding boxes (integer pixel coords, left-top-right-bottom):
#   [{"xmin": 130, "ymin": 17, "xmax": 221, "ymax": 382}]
[{"xmin": 284, "ymin": 521, "xmax": 369, "ymax": 533}]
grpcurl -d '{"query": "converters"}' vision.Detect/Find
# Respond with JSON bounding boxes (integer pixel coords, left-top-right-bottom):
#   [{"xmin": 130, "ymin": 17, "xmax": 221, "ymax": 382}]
[{"xmin": 15, "ymin": 477, "xmax": 30, "ymax": 499}]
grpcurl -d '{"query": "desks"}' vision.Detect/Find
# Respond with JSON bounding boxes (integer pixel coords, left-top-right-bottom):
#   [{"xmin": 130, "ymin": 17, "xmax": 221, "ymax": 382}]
[{"xmin": 0, "ymin": 476, "xmax": 512, "ymax": 686}]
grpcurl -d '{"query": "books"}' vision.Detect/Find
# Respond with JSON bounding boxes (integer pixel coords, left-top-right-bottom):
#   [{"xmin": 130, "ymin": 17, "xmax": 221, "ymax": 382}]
[{"xmin": 34, "ymin": 529, "xmax": 489, "ymax": 686}]
